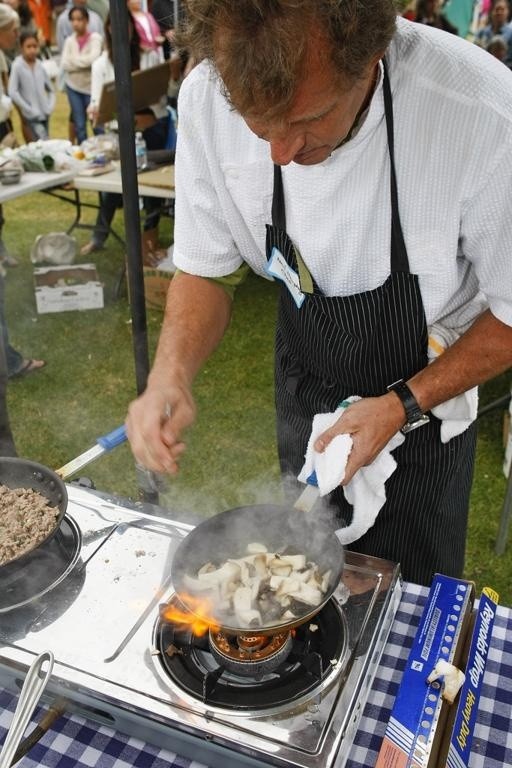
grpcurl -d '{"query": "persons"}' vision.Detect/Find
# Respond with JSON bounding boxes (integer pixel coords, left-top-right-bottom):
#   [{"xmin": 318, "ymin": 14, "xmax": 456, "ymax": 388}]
[
  {"xmin": 396, "ymin": 0, "xmax": 512, "ymax": 67},
  {"xmin": 0, "ymin": 0, "xmax": 205, "ymax": 281},
  {"xmin": 0, "ymin": 279, "xmax": 45, "ymax": 379},
  {"xmin": 121, "ymin": 0, "xmax": 512, "ymax": 585}
]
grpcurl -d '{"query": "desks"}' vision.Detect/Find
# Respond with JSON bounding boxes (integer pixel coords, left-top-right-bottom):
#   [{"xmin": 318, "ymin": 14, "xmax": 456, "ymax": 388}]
[
  {"xmin": 1, "ymin": 584, "xmax": 512, "ymax": 768},
  {"xmin": 0, "ymin": 161, "xmax": 172, "ymax": 304}
]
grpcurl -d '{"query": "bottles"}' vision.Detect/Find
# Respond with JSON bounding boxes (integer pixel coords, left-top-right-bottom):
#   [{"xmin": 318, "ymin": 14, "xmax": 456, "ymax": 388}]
[{"xmin": 136, "ymin": 132, "xmax": 148, "ymax": 173}]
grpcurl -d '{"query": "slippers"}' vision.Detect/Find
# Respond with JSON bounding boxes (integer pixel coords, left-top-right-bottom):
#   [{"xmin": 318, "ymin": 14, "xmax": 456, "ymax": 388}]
[{"xmin": 9, "ymin": 359, "xmax": 46, "ymax": 380}]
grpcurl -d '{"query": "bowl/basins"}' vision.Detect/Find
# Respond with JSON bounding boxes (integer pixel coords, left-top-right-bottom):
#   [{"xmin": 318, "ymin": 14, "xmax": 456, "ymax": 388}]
[{"xmin": 1, "ymin": 168, "xmax": 21, "ymax": 185}]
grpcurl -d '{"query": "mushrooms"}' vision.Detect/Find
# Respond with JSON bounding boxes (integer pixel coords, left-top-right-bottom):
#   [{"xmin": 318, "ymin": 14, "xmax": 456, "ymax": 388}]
[{"xmin": 183, "ymin": 547, "xmax": 331, "ymax": 629}]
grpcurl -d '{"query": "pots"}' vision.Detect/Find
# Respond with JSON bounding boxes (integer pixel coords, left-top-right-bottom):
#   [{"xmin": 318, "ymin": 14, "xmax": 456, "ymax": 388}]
[
  {"xmin": 0, "ymin": 420, "xmax": 128, "ymax": 570},
  {"xmin": 170, "ymin": 470, "xmax": 347, "ymax": 633}
]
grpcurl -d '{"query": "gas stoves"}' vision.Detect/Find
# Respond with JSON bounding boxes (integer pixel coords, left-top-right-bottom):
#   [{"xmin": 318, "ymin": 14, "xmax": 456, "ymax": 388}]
[{"xmin": 0, "ymin": 481, "xmax": 406, "ymax": 766}]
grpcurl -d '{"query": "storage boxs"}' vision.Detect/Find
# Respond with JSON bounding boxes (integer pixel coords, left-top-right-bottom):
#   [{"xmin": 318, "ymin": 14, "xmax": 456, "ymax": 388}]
[{"xmin": 35, "ymin": 230, "xmax": 173, "ymax": 314}]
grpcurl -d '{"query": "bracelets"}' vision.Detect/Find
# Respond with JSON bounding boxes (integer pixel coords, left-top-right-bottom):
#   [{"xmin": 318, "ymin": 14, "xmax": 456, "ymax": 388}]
[{"xmin": 387, "ymin": 378, "xmax": 432, "ymax": 435}]
